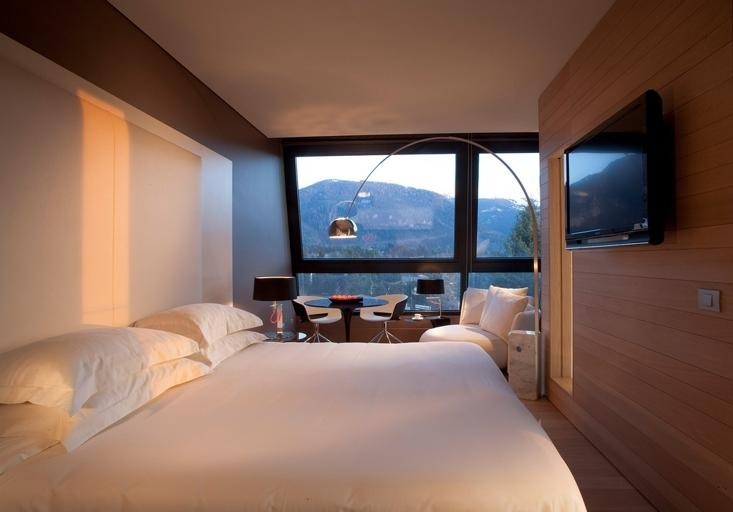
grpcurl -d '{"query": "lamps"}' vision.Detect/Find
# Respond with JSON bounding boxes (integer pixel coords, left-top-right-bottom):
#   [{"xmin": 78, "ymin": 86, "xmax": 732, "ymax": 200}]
[
  {"xmin": 416, "ymin": 278, "xmax": 451, "ymax": 319},
  {"xmin": 328, "ymin": 137, "xmax": 546, "ymax": 400},
  {"xmin": 253, "ymin": 275, "xmax": 297, "ymax": 340}
]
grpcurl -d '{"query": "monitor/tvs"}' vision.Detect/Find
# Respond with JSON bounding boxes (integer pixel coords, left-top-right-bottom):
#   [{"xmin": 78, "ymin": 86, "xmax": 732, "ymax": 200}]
[{"xmin": 564, "ymin": 89, "xmax": 677, "ymax": 249}]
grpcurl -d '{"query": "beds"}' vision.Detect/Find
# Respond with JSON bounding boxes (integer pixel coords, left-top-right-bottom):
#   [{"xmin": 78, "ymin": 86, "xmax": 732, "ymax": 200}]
[{"xmin": 0, "ymin": 342, "xmax": 587, "ymax": 512}]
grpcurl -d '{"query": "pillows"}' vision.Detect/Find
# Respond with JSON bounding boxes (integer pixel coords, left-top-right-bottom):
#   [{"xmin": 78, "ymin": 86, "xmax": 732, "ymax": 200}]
[{"xmin": 459, "ymin": 287, "xmax": 530, "ymax": 344}]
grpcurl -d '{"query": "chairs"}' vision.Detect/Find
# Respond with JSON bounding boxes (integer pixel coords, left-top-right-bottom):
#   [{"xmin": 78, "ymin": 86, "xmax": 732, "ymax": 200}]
[
  {"xmin": 292, "ymin": 293, "xmax": 409, "ymax": 344},
  {"xmin": 409, "ymin": 285, "xmax": 541, "ymax": 368}
]
